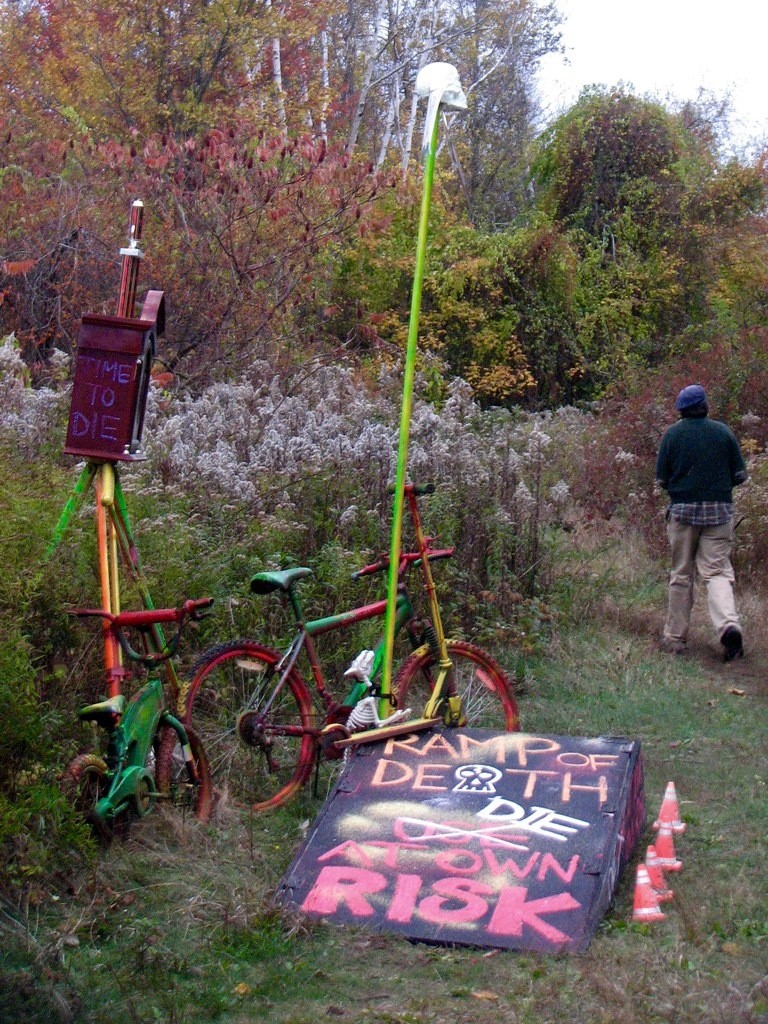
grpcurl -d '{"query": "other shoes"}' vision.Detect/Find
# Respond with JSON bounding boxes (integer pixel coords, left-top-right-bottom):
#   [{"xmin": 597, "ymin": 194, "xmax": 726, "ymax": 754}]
[
  {"xmin": 720, "ymin": 622, "xmax": 745, "ymax": 663},
  {"xmin": 657, "ymin": 638, "xmax": 686, "ymax": 654}
]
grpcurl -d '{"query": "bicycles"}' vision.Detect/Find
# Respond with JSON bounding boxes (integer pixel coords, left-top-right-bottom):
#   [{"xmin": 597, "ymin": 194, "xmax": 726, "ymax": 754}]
[
  {"xmin": 59, "ymin": 597, "xmax": 214, "ymax": 859},
  {"xmin": 182, "ymin": 544, "xmax": 521, "ymax": 810}
]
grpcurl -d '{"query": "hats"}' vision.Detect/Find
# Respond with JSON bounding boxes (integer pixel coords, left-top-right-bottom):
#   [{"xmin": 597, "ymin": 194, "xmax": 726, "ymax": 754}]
[{"xmin": 676, "ymin": 385, "xmax": 705, "ymax": 409}]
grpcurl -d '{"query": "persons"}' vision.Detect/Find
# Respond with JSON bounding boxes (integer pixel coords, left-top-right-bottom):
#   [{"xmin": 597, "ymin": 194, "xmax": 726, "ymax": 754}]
[{"xmin": 655, "ymin": 385, "xmax": 749, "ymax": 662}]
